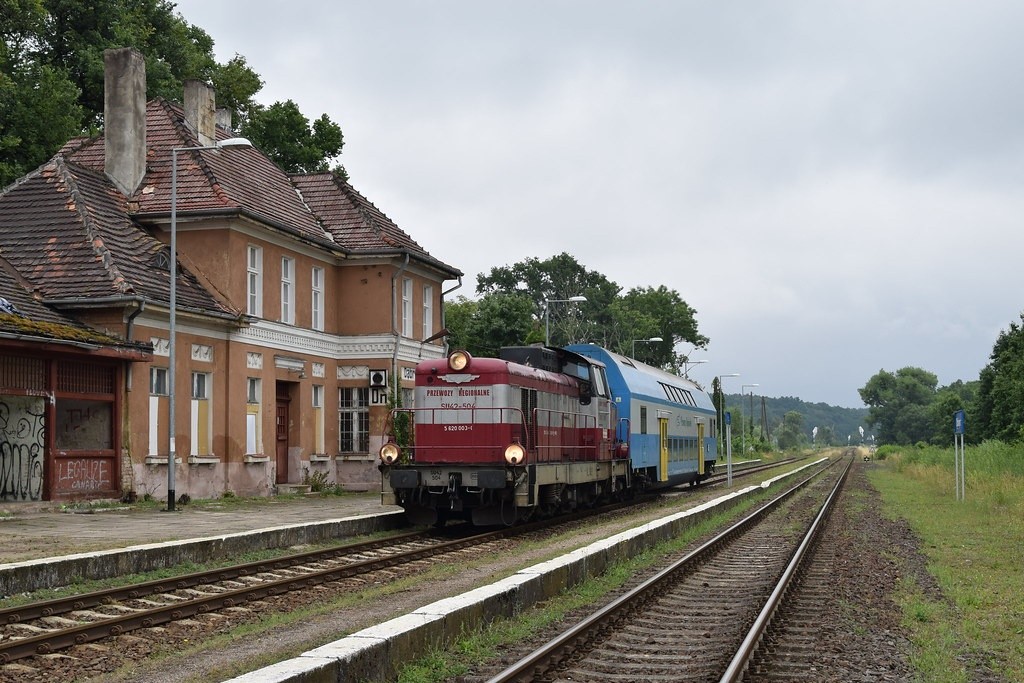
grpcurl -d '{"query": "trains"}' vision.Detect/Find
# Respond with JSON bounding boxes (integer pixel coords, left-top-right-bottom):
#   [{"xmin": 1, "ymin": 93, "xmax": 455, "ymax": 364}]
[{"xmin": 378, "ymin": 328, "xmax": 718, "ymax": 534}]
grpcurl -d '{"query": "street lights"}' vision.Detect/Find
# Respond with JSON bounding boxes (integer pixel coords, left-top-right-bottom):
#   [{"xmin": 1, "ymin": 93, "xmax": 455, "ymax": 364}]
[
  {"xmin": 743, "ymin": 384, "xmax": 760, "ymax": 457},
  {"xmin": 720, "ymin": 374, "xmax": 741, "ymax": 459},
  {"xmin": 632, "ymin": 338, "xmax": 663, "ymax": 360},
  {"xmin": 543, "ymin": 296, "xmax": 587, "ymax": 347},
  {"xmin": 685, "ymin": 360, "xmax": 708, "ymax": 380},
  {"xmin": 168, "ymin": 138, "xmax": 254, "ymax": 511}
]
